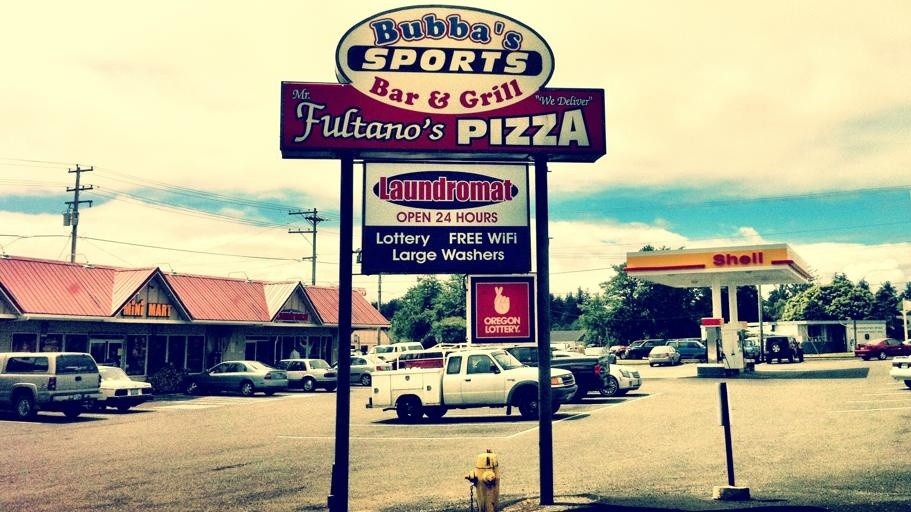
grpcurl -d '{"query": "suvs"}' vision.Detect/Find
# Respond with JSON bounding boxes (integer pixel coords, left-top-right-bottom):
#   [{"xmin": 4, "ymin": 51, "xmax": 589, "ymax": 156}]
[
  {"xmin": 615, "ymin": 340, "xmax": 644, "ymax": 360},
  {"xmin": 763, "ymin": 335, "xmax": 805, "ymax": 363},
  {"xmin": 743, "ymin": 339, "xmax": 761, "ymax": 362}
]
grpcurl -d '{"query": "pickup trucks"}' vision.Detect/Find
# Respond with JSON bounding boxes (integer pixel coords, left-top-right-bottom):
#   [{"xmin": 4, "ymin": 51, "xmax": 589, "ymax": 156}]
[
  {"xmin": 362, "ymin": 343, "xmax": 642, "ymax": 414},
  {"xmin": 625, "ymin": 339, "xmax": 666, "ymax": 359}
]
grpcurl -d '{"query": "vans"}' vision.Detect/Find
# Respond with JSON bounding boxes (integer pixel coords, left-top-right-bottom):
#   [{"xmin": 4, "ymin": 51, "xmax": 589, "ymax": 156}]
[
  {"xmin": 0, "ymin": 351, "xmax": 102, "ymax": 420},
  {"xmin": 664, "ymin": 340, "xmax": 706, "ymax": 363}
]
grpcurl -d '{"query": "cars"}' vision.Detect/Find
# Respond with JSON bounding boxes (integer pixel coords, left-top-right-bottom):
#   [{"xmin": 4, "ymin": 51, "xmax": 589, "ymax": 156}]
[
  {"xmin": 648, "ymin": 345, "xmax": 681, "ymax": 367},
  {"xmin": 902, "ymin": 339, "xmax": 911, "ymax": 345},
  {"xmin": 331, "ymin": 353, "xmax": 391, "ymax": 386},
  {"xmin": 430, "ymin": 341, "xmax": 475, "ymax": 349},
  {"xmin": 275, "ymin": 357, "xmax": 337, "ymax": 391},
  {"xmin": 185, "ymin": 359, "xmax": 288, "ymax": 395},
  {"xmin": 81, "ymin": 365, "xmax": 152, "ymax": 413},
  {"xmin": 889, "ymin": 355, "xmax": 911, "ymax": 388},
  {"xmin": 853, "ymin": 337, "xmax": 910, "ymax": 360}
]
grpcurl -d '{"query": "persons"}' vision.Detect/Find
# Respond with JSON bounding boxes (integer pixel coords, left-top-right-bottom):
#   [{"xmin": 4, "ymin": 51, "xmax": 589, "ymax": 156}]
[{"xmin": 288, "ymin": 346, "xmax": 302, "ymax": 358}]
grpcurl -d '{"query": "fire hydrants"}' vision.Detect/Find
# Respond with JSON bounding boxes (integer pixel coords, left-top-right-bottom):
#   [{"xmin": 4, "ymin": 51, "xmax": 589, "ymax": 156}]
[{"xmin": 462, "ymin": 446, "xmax": 502, "ymax": 512}]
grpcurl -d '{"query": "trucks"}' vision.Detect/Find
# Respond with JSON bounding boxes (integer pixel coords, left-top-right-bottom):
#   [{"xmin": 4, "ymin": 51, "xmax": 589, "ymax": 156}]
[{"xmin": 368, "ymin": 338, "xmax": 424, "ymax": 363}]
